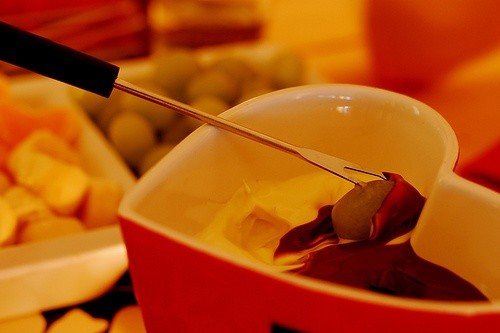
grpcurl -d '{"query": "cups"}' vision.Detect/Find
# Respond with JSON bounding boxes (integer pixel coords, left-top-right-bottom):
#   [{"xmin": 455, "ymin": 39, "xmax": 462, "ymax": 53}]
[{"xmin": 118, "ymin": 82, "xmax": 500, "ymax": 333}]
